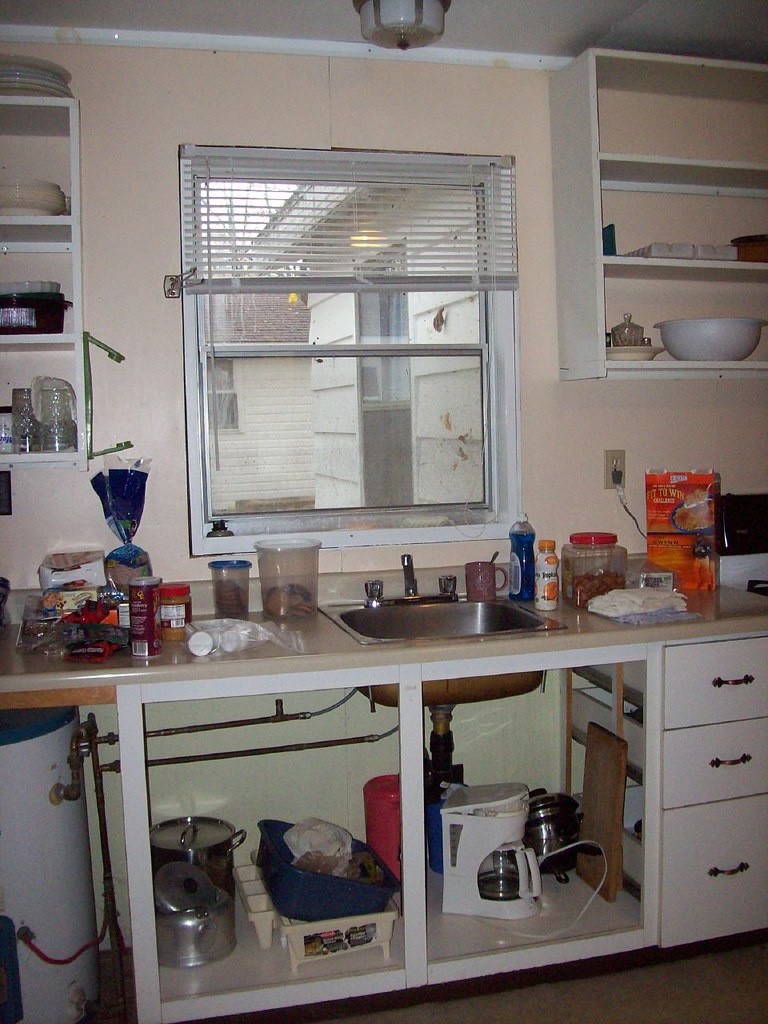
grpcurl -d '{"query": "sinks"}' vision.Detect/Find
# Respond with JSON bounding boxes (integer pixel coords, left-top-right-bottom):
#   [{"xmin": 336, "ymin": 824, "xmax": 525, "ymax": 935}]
[{"xmin": 338, "ymin": 602, "xmax": 547, "ymax": 706}]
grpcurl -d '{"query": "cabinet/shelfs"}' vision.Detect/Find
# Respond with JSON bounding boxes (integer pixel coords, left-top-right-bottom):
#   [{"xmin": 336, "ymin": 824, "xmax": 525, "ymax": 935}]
[
  {"xmin": 549, "ymin": 47, "xmax": 768, "ymax": 383},
  {"xmin": 0, "ymin": 98, "xmax": 92, "ymax": 468},
  {"xmin": 573, "ymin": 636, "xmax": 768, "ymax": 948}
]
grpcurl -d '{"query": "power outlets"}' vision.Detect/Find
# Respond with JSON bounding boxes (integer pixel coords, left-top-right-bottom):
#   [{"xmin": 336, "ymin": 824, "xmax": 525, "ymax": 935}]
[{"xmin": 603, "ymin": 448, "xmax": 626, "ymax": 491}]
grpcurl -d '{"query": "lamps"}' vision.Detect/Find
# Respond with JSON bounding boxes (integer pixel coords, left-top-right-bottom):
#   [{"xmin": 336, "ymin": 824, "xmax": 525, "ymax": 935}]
[
  {"xmin": 351, "ymin": 217, "xmax": 387, "ymax": 249},
  {"xmin": 350, "ymin": 0, "xmax": 453, "ymax": 54}
]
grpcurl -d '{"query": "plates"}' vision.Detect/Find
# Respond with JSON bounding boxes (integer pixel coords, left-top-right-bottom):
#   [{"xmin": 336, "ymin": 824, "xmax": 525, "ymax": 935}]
[
  {"xmin": 0, "ymin": 53, "xmax": 72, "ymax": 98},
  {"xmin": 606, "ymin": 346, "xmax": 666, "ymax": 361},
  {"xmin": 0, "ymin": 178, "xmax": 67, "ymax": 215}
]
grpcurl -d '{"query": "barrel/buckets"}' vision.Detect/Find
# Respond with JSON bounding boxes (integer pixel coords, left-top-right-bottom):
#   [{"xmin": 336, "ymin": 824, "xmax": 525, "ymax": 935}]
[
  {"xmin": 363, "ymin": 774, "xmax": 401, "ymax": 893},
  {"xmin": 149, "ymin": 816, "xmax": 247, "ymax": 901}
]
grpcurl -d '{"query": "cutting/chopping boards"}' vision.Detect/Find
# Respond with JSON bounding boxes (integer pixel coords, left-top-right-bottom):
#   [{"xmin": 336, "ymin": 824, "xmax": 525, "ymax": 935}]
[{"xmin": 575, "ymin": 721, "xmax": 628, "ymax": 903}]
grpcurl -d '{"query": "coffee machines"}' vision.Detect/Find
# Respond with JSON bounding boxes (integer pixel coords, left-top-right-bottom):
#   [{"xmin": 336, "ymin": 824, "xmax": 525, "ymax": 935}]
[{"xmin": 439, "ymin": 782, "xmax": 541, "ymax": 919}]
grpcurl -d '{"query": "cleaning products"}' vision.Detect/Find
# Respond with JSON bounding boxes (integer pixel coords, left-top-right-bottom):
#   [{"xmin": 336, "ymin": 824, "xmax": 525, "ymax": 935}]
[{"xmin": 508, "ymin": 513, "xmax": 537, "ymax": 602}]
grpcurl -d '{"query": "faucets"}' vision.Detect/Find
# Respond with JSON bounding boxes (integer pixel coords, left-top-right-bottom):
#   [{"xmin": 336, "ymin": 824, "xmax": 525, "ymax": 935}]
[{"xmin": 401, "ymin": 553, "xmax": 418, "ymax": 597}]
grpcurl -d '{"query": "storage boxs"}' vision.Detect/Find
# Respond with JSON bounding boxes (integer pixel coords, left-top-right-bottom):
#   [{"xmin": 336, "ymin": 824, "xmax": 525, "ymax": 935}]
[
  {"xmin": 646, "ymin": 472, "xmax": 721, "ymax": 590},
  {"xmin": 39, "ymin": 551, "xmax": 106, "ymax": 592},
  {"xmin": 256, "ymin": 819, "xmax": 400, "ymax": 923}
]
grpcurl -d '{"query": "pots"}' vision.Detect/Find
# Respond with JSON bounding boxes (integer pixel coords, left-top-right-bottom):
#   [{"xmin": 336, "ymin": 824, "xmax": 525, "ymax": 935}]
[{"xmin": 0, "ymin": 292, "xmax": 73, "ymax": 336}]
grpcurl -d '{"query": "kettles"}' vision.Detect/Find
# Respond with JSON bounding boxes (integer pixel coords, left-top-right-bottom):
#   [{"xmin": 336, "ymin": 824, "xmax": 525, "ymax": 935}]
[{"xmin": 154, "ymin": 861, "xmax": 237, "ymax": 970}]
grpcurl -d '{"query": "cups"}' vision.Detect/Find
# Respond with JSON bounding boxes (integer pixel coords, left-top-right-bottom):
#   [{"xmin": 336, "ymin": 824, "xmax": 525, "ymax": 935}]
[
  {"xmin": 465, "ymin": 562, "xmax": 508, "ymax": 602},
  {"xmin": 208, "ymin": 560, "xmax": 252, "ymax": 621},
  {"xmin": 253, "ymin": 538, "xmax": 322, "ymax": 622},
  {"xmin": 12, "ymin": 388, "xmax": 41, "ymax": 453},
  {"xmin": 40, "ymin": 387, "xmax": 78, "ymax": 453}
]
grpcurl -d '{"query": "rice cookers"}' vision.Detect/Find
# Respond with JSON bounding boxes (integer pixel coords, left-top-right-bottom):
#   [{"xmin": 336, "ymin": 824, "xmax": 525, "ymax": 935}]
[{"xmin": 522, "ymin": 788, "xmax": 584, "ymax": 874}]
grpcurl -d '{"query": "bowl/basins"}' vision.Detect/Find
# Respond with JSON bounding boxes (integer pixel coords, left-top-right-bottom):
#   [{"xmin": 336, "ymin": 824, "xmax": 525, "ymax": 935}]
[
  {"xmin": 670, "ymin": 494, "xmax": 713, "ymax": 534},
  {"xmin": 257, "ymin": 818, "xmax": 400, "ymax": 923},
  {"xmin": 653, "ymin": 317, "xmax": 768, "ymax": 361},
  {"xmin": 730, "ymin": 234, "xmax": 768, "ymax": 262}
]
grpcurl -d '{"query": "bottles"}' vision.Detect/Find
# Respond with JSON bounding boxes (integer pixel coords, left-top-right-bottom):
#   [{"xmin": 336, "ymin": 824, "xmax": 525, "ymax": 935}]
[
  {"xmin": 534, "ymin": 540, "xmax": 559, "ymax": 611},
  {"xmin": 611, "ymin": 313, "xmax": 652, "ymax": 347},
  {"xmin": 561, "ymin": 532, "xmax": 627, "ymax": 609},
  {"xmin": 509, "ymin": 513, "xmax": 536, "ymax": 602},
  {"xmin": 0, "ymin": 406, "xmax": 12, "ymax": 454}
]
